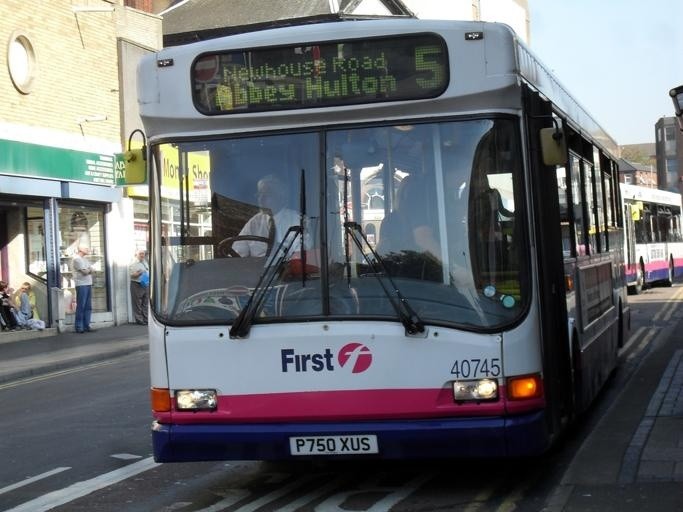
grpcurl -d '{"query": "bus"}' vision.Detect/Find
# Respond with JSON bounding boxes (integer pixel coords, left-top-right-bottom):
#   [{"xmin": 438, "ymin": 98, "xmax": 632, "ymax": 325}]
[
  {"xmin": 618, "ymin": 183, "xmax": 683, "ymax": 295},
  {"xmin": 125, "ymin": 20, "xmax": 631, "ymax": 463}
]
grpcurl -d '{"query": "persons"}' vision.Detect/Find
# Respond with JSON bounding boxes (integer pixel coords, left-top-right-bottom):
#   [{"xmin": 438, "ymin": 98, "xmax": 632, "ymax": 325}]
[
  {"xmin": 374, "ymin": 160, "xmax": 517, "ymax": 281},
  {"xmin": 71, "ymin": 243, "xmax": 96, "ymax": 333},
  {"xmin": 129, "ymin": 248, "xmax": 150, "ymax": 325},
  {"xmin": 227, "ymin": 177, "xmax": 316, "ymax": 261},
  {"xmin": 0, "ymin": 281, "xmax": 46, "ymax": 332}
]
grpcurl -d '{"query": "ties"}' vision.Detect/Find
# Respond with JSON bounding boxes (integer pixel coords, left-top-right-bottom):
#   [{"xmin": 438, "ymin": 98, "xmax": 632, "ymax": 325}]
[{"xmin": 265, "ymin": 218, "xmax": 275, "ymax": 257}]
